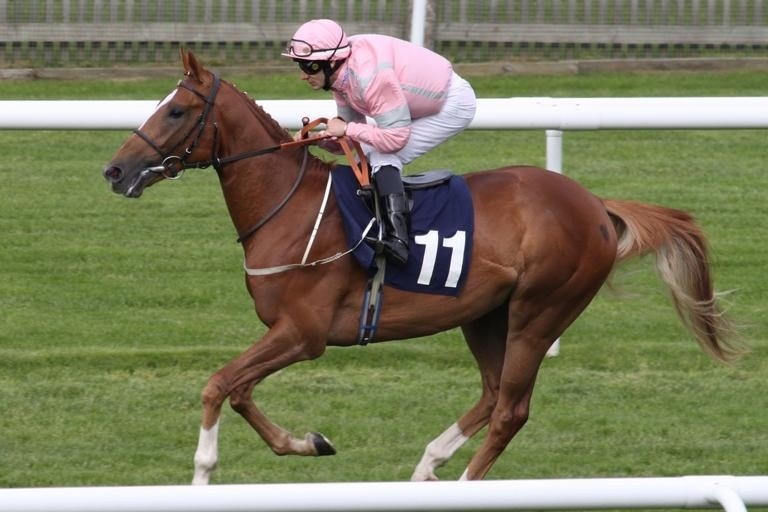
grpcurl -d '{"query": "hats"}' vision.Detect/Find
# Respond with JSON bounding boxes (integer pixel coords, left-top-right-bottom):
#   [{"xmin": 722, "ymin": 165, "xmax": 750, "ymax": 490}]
[{"xmin": 281, "ymin": 19, "xmax": 350, "ymax": 61}]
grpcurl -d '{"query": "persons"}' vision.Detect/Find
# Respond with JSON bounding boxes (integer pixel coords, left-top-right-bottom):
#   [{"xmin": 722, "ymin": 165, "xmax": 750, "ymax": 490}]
[{"xmin": 280, "ymin": 18, "xmax": 475, "ymax": 267}]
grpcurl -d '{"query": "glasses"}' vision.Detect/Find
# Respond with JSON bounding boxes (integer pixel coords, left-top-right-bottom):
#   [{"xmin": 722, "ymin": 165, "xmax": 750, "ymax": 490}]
[{"xmin": 292, "ymin": 58, "xmax": 323, "ymax": 75}]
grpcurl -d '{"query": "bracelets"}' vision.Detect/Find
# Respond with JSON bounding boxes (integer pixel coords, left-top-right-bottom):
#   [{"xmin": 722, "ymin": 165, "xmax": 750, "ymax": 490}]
[{"xmin": 343, "ymin": 121, "xmax": 349, "ymax": 135}]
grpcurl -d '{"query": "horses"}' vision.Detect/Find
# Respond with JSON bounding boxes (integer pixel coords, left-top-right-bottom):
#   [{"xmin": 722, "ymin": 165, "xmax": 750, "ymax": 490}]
[{"xmin": 100, "ymin": 43, "xmax": 755, "ymax": 486}]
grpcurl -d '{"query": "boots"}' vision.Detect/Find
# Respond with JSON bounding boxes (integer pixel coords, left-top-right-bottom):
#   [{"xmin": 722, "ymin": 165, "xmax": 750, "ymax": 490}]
[{"xmin": 365, "ymin": 192, "xmax": 410, "ymax": 264}]
